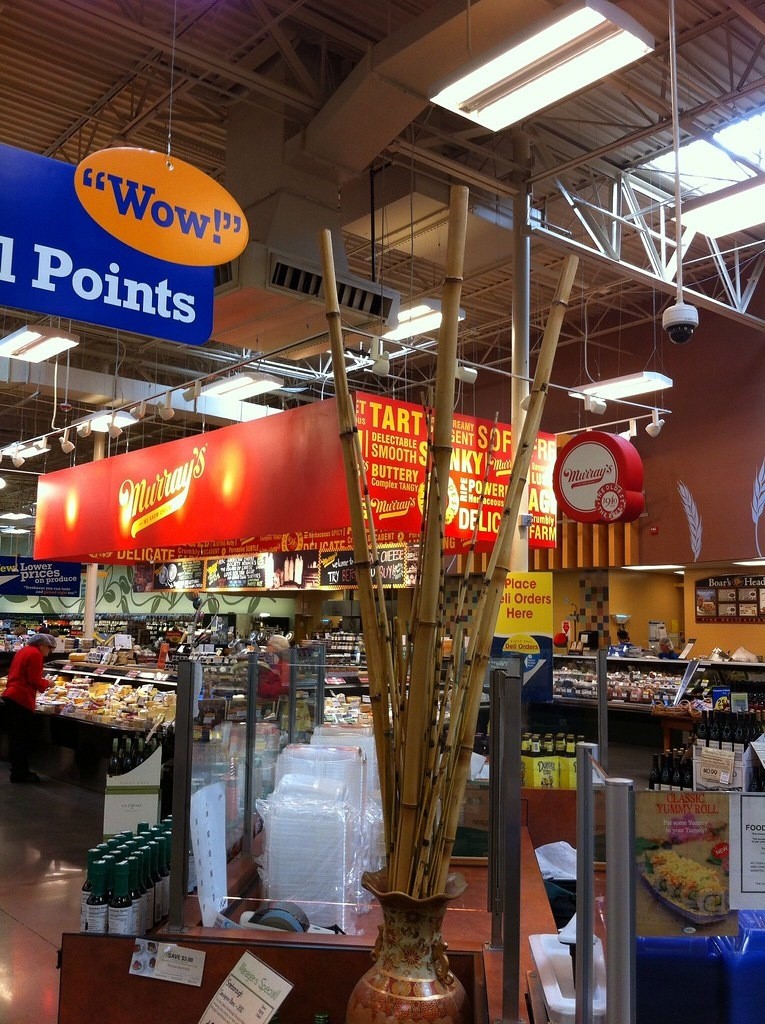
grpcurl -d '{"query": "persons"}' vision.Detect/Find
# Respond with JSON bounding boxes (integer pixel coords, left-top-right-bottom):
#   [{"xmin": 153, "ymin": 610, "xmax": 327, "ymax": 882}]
[
  {"xmin": 658, "ymin": 637, "xmax": 679, "ymax": 660},
  {"xmin": 38, "ymin": 623, "xmax": 50, "ymax": 634},
  {"xmin": 258, "ymin": 634, "xmax": 290, "ymax": 720},
  {"xmin": 12, "ymin": 623, "xmax": 28, "ymax": 636},
  {"xmin": 616, "ymin": 630, "xmax": 633, "ymax": 647},
  {"xmin": 0, "ymin": 633, "xmax": 57, "ymax": 783},
  {"xmin": 2, "ymin": 623, "xmax": 11, "ymax": 635}
]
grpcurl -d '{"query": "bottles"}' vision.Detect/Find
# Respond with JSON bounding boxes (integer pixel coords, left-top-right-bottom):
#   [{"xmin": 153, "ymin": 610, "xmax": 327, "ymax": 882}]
[
  {"xmin": 697, "ymin": 708, "xmax": 765, "ymax": 753},
  {"xmin": 520, "ymin": 732, "xmax": 587, "ymax": 755},
  {"xmin": 648, "ymin": 747, "xmax": 694, "ymax": 792},
  {"xmin": 80, "ymin": 814, "xmax": 173, "ymax": 936},
  {"xmin": 607, "ymin": 681, "xmax": 677, "ymax": 707}
]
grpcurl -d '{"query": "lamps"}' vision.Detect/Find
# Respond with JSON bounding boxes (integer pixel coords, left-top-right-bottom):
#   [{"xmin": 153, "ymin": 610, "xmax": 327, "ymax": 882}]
[
  {"xmin": 156, "ymin": 391, "xmax": 174, "ymax": 421},
  {"xmin": 2, "ymin": 440, "xmax": 51, "ymax": 458},
  {"xmin": 71, "ymin": 410, "xmax": 139, "ymax": 432},
  {"xmin": 130, "ymin": 401, "xmax": 146, "ymax": 419},
  {"xmin": 520, "ymin": 395, "xmax": 532, "ymax": 411},
  {"xmin": 182, "ymin": 382, "xmax": 201, "ymax": 402},
  {"xmin": 11, "ymin": 453, "xmax": 25, "ymax": 466},
  {"xmin": 59, "ymin": 429, "xmax": 75, "ymax": 454},
  {"xmin": 428, "ymin": 0, "xmax": 655, "ymax": 133},
  {"xmin": 107, "ymin": 412, "xmax": 123, "ymax": 439},
  {"xmin": 567, "ymin": 372, "xmax": 673, "ymax": 441},
  {"xmin": 372, "ymin": 298, "xmax": 477, "ymax": 384},
  {"xmin": 77, "ymin": 419, "xmax": 92, "ymax": 438},
  {"xmin": 0, "ymin": 308, "xmax": 81, "ymax": 364},
  {"xmin": 670, "ymin": 173, "xmax": 765, "ymax": 239},
  {"xmin": 201, "ymin": 372, "xmax": 284, "ymax": 403}
]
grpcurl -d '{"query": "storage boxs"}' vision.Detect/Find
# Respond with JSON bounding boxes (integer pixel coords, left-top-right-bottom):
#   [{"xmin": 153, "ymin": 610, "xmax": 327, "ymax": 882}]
[
  {"xmin": 528, "ymin": 909, "xmax": 765, "ymax": 1024},
  {"xmin": 693, "ymin": 732, "xmax": 765, "ymax": 793}
]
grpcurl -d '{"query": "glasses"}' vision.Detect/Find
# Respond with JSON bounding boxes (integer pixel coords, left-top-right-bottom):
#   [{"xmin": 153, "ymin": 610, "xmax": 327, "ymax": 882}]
[{"xmin": 49, "ymin": 646, "xmax": 52, "ymax": 655}]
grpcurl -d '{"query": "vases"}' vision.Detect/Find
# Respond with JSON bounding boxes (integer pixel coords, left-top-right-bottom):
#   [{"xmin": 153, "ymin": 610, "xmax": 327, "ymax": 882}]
[{"xmin": 345, "ymin": 866, "xmax": 467, "ymax": 1024}]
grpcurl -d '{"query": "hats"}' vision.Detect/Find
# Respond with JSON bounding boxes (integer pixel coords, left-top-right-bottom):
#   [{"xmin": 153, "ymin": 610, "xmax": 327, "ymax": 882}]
[{"xmin": 27, "ymin": 634, "xmax": 57, "ymax": 649}]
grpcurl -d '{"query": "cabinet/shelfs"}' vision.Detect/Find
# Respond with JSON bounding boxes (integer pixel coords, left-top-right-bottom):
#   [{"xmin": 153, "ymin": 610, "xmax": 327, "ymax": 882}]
[{"xmin": 57, "ymin": 819, "xmax": 559, "ymax": 1024}]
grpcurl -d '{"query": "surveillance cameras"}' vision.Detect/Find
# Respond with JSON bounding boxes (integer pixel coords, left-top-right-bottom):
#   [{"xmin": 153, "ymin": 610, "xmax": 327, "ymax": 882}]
[{"xmin": 663, "ymin": 303, "xmax": 698, "ymax": 344}]
